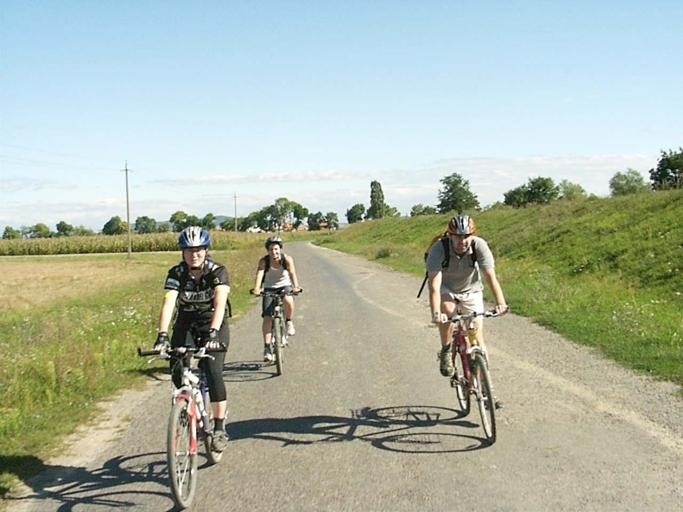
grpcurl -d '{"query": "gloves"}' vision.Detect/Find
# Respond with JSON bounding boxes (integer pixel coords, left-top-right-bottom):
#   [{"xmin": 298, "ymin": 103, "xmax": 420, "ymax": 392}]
[
  {"xmin": 205, "ymin": 329, "xmax": 221, "ymax": 350},
  {"xmin": 152, "ymin": 331, "xmax": 171, "ymax": 357}
]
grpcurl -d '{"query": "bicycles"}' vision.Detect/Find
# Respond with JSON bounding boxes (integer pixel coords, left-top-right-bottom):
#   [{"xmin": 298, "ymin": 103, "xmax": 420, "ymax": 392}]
[
  {"xmin": 135, "ymin": 344, "xmax": 228, "ymax": 509},
  {"xmin": 427, "ymin": 303, "xmax": 512, "ymax": 443},
  {"xmin": 249, "ymin": 287, "xmax": 303, "ymax": 375}
]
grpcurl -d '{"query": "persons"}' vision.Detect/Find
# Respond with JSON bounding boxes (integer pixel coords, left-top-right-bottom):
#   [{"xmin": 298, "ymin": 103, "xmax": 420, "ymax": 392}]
[
  {"xmin": 251, "ymin": 235, "xmax": 297, "ymax": 362},
  {"xmin": 423, "ymin": 213, "xmax": 508, "ymax": 412},
  {"xmin": 153, "ymin": 224, "xmax": 233, "ymax": 455}
]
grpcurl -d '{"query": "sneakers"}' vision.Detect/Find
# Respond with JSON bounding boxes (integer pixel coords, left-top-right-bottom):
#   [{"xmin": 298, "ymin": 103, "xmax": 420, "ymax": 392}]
[
  {"xmin": 262, "ymin": 347, "xmax": 273, "ymax": 362},
  {"xmin": 438, "ymin": 348, "xmax": 455, "ymax": 377},
  {"xmin": 484, "ymin": 389, "xmax": 504, "ymax": 410},
  {"xmin": 284, "ymin": 320, "xmax": 295, "ymax": 335},
  {"xmin": 211, "ymin": 429, "xmax": 229, "ymax": 453}
]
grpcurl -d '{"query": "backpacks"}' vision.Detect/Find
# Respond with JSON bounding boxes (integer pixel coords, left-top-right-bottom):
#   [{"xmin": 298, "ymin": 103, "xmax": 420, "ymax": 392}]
[{"xmin": 424, "ymin": 234, "xmax": 478, "ymax": 275}]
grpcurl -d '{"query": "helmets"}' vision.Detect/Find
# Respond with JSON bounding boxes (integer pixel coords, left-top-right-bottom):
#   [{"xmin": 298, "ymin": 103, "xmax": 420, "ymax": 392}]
[
  {"xmin": 179, "ymin": 226, "xmax": 211, "ymax": 248},
  {"xmin": 448, "ymin": 214, "xmax": 476, "ymax": 236},
  {"xmin": 265, "ymin": 237, "xmax": 283, "ymax": 248}
]
